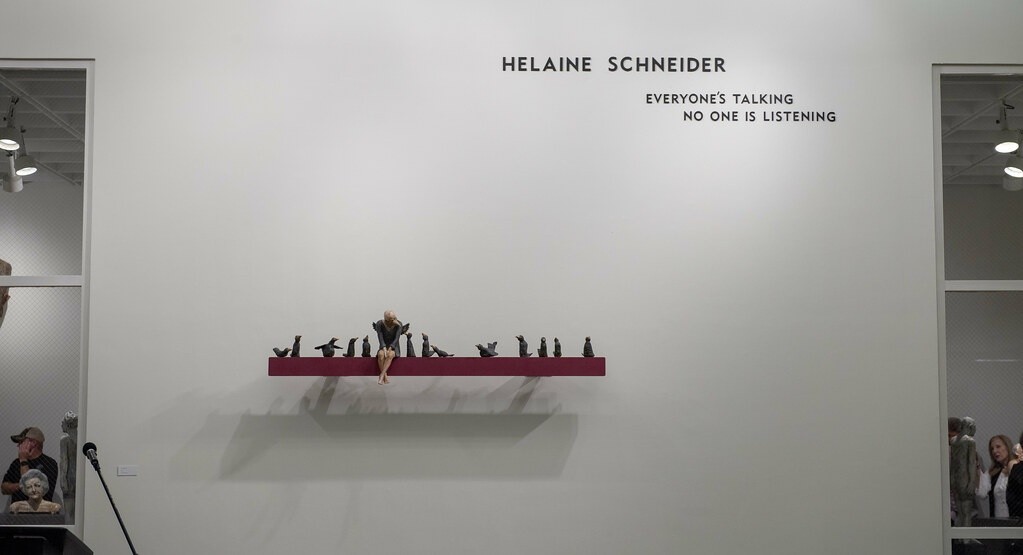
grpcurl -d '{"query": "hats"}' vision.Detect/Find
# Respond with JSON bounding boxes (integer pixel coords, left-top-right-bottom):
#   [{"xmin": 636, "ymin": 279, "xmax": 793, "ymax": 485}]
[
  {"xmin": 11, "ymin": 427, "xmax": 44, "ymax": 443},
  {"xmin": 949, "ymin": 417, "xmax": 961, "ymax": 433}
]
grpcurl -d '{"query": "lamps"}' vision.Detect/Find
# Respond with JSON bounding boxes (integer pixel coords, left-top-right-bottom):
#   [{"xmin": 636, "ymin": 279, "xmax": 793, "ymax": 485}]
[
  {"xmin": 14, "ymin": 125, "xmax": 38, "ymax": 177},
  {"xmin": 0, "ymin": 95, "xmax": 21, "ymax": 150},
  {"xmin": 3, "ymin": 152, "xmax": 24, "ymax": 193},
  {"xmin": 994, "ymin": 98, "xmax": 1020, "ymax": 153},
  {"xmin": 1003, "ymin": 129, "xmax": 1023, "ymax": 179}
]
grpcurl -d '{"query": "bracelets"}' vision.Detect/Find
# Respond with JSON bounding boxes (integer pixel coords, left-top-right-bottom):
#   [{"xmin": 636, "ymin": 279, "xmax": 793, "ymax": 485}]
[{"xmin": 20, "ymin": 461, "xmax": 28, "ymax": 466}]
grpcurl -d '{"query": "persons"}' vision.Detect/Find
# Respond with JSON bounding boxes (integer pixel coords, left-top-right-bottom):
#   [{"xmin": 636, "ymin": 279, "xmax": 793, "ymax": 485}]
[
  {"xmin": 57, "ymin": 411, "xmax": 78, "ymax": 527},
  {"xmin": 950, "ymin": 415, "xmax": 985, "ymax": 543},
  {"xmin": 986, "ymin": 434, "xmax": 1022, "ymax": 520},
  {"xmin": 376, "ymin": 310, "xmax": 403, "ymax": 384},
  {"xmin": 948, "ymin": 418, "xmax": 989, "ymax": 529},
  {"xmin": 10, "ymin": 468, "xmax": 61, "ymax": 514},
  {"xmin": 0, "ymin": 426, "xmax": 57, "ymax": 512}
]
